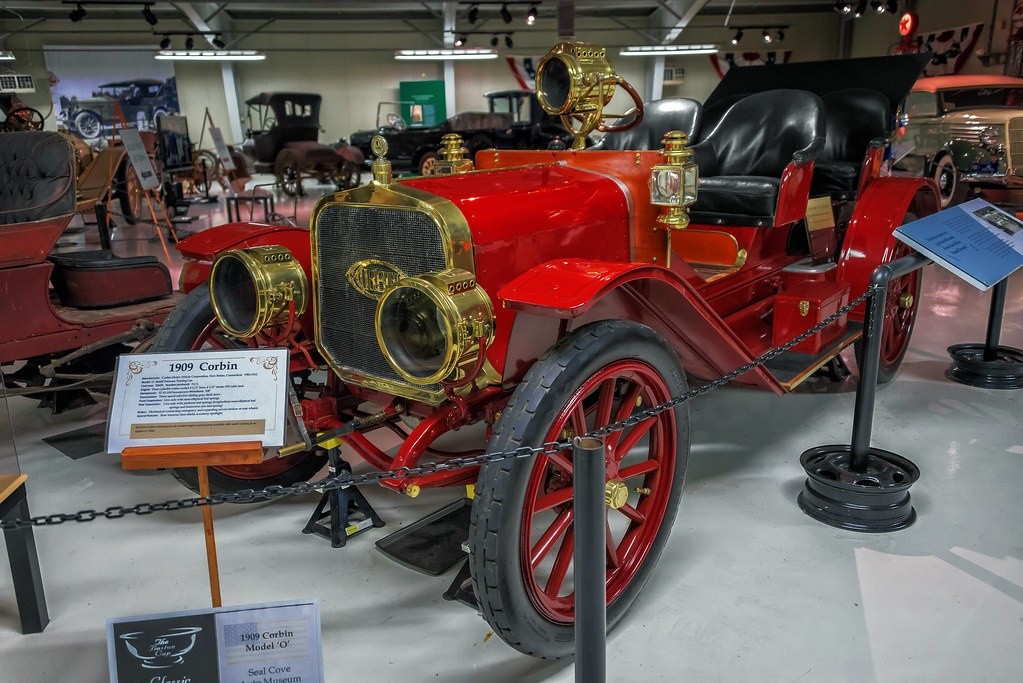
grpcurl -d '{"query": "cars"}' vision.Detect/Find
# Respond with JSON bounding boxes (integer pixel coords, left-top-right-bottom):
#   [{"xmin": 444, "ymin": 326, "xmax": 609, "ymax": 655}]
[
  {"xmin": 350, "ymin": 100, "xmax": 427, "ymax": 180},
  {"xmin": 63, "ymin": 77, "xmax": 166, "ymax": 140},
  {"xmin": 406, "ymin": 92, "xmax": 551, "ymax": 175},
  {"xmin": 131, "ymin": 50, "xmax": 942, "ymax": 663},
  {"xmin": 882, "ymin": 75, "xmax": 1023, "ymax": 212}
]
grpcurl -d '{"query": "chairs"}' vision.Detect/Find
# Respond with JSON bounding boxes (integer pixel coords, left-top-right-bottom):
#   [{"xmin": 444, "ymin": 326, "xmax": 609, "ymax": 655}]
[
  {"xmin": 585, "ymin": 97, "xmax": 702, "ymax": 150},
  {"xmin": 48, "ymin": 248, "xmax": 173, "ymax": 307},
  {"xmin": 685, "ymin": 89, "xmax": 827, "ymax": 228},
  {"xmin": 0, "ymin": 132, "xmax": 77, "ymax": 268}
]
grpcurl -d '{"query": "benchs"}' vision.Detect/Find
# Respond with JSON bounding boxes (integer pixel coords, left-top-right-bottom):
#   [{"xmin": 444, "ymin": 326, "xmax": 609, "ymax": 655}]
[{"xmin": 686, "ymin": 89, "xmax": 890, "ymax": 225}]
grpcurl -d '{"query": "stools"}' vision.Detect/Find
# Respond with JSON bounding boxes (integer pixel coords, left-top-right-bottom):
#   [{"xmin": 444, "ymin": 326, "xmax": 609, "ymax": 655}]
[{"xmin": 225, "ymin": 190, "xmax": 275, "ymax": 225}]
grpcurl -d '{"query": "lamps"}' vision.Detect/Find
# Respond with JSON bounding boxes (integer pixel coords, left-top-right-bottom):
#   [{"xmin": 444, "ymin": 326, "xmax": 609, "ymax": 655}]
[
  {"xmin": 159, "ymin": 35, "xmax": 171, "ymax": 50},
  {"xmin": 775, "ymin": 30, "xmax": 785, "ymax": 42},
  {"xmin": 617, "ymin": 43, "xmax": 720, "ymax": 56},
  {"xmin": 142, "ymin": 5, "xmax": 158, "ymax": 26},
  {"xmin": 394, "ymin": 49, "xmax": 499, "ymax": 60},
  {"xmin": 69, "ymin": 9, "xmax": 87, "ymax": 22},
  {"xmin": 835, "ymin": 0, "xmax": 886, "ymax": 19},
  {"xmin": 454, "ymin": 35, "xmax": 468, "ymax": 47},
  {"xmin": 468, "ymin": 6, "xmax": 479, "ymax": 25},
  {"xmin": 505, "ymin": 36, "xmax": 513, "ymax": 49},
  {"xmin": 525, "ymin": 7, "xmax": 538, "ymax": 26},
  {"xmin": 760, "ymin": 31, "xmax": 772, "ymax": 43},
  {"xmin": 153, "ymin": 50, "xmax": 266, "ymax": 60},
  {"xmin": 731, "ymin": 30, "xmax": 744, "ymax": 45},
  {"xmin": 185, "ymin": 35, "xmax": 193, "ymax": 50},
  {"xmin": 212, "ymin": 35, "xmax": 225, "ymax": 49},
  {"xmin": 490, "ymin": 37, "xmax": 498, "ymax": 47}
]
